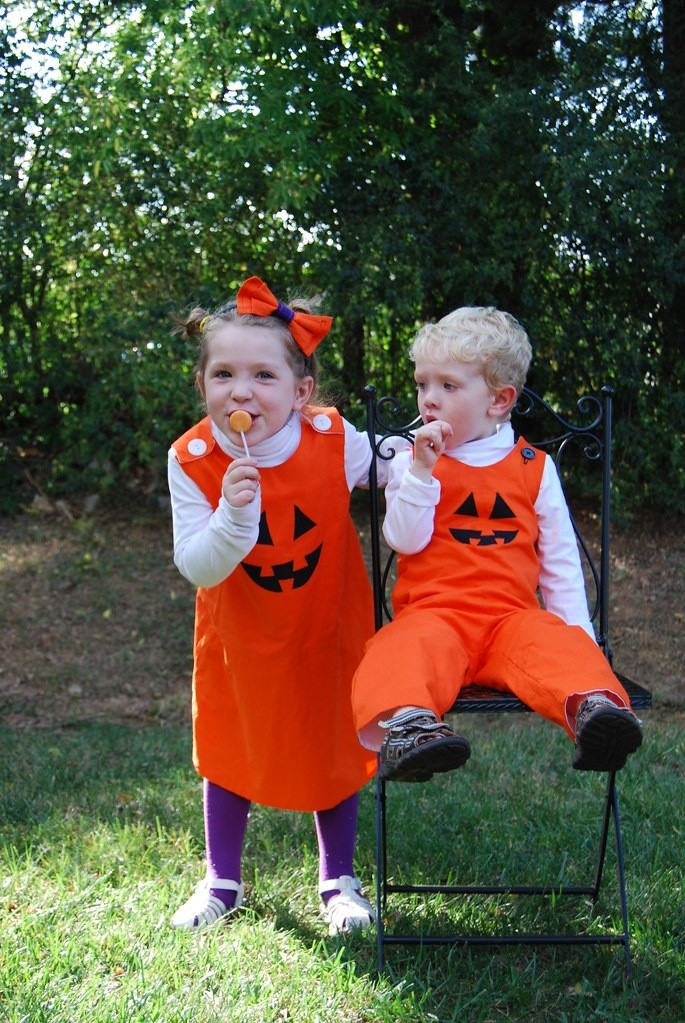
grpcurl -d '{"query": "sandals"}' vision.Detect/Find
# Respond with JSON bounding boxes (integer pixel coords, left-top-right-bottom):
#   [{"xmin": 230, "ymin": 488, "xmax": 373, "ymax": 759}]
[
  {"xmin": 318, "ymin": 875, "xmax": 376, "ymax": 934},
  {"xmin": 572, "ymin": 695, "xmax": 643, "ymax": 772},
  {"xmin": 170, "ymin": 877, "xmax": 244, "ymax": 930},
  {"xmin": 377, "ymin": 708, "xmax": 470, "ymax": 783}
]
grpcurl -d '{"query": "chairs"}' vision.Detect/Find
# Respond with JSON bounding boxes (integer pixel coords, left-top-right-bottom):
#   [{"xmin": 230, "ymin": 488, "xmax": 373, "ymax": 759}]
[{"xmin": 360, "ymin": 380, "xmax": 651, "ymax": 971}]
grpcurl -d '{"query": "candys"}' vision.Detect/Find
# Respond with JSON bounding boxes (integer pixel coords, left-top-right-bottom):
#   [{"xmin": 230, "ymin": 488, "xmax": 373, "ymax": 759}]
[{"xmin": 230, "ymin": 409, "xmax": 252, "ymax": 458}]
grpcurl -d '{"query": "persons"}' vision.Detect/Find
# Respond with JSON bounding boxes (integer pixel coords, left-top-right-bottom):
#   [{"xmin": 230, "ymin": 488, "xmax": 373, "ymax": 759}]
[
  {"xmin": 167, "ymin": 274, "xmax": 420, "ymax": 941},
  {"xmin": 350, "ymin": 305, "xmax": 645, "ymax": 783}
]
